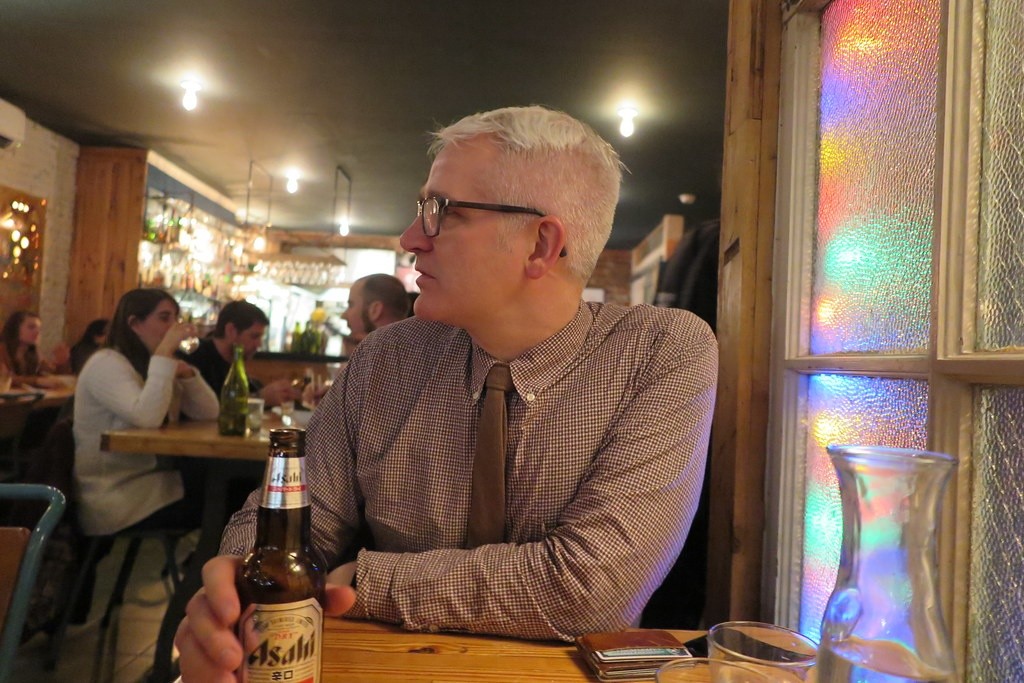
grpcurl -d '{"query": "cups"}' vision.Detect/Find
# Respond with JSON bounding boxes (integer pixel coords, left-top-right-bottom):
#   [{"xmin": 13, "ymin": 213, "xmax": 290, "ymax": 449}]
[
  {"xmin": 246, "ymin": 397, "xmax": 264, "ymax": 430},
  {"xmin": 178, "ymin": 335, "xmax": 200, "ymax": 353},
  {"xmin": 655, "ymin": 657, "xmax": 770, "ymax": 683},
  {"xmin": 706, "ymin": 621, "xmax": 817, "ymax": 683},
  {"xmin": 280, "ymin": 400, "xmax": 295, "ymax": 424}
]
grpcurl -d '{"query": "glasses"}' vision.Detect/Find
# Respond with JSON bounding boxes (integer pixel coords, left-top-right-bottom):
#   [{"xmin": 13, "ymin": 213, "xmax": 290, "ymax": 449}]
[{"xmin": 417, "ymin": 194, "xmax": 568, "ymax": 258}]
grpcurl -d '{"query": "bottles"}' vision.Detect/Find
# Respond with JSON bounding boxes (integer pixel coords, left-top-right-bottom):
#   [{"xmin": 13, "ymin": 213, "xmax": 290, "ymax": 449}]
[
  {"xmin": 218, "ymin": 343, "xmax": 249, "ymax": 435},
  {"xmin": 235, "ymin": 426, "xmax": 326, "ymax": 682},
  {"xmin": 817, "ymin": 444, "xmax": 962, "ymax": 683},
  {"xmin": 138, "ymin": 204, "xmax": 232, "ymax": 325},
  {"xmin": 291, "ymin": 300, "xmax": 327, "ymax": 353}
]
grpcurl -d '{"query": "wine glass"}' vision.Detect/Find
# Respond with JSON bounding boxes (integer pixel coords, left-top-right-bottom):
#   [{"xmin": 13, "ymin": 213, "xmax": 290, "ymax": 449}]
[{"xmin": 252, "ymin": 260, "xmax": 345, "ymax": 286}]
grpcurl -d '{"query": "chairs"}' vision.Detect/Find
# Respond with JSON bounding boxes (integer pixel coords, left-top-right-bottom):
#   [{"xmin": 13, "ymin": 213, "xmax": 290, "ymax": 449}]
[{"xmin": 0, "ymin": 391, "xmax": 197, "ymax": 683}]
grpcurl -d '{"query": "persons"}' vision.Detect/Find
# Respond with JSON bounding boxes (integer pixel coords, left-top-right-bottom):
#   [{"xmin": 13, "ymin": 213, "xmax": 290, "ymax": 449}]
[
  {"xmin": 299, "ymin": 274, "xmax": 420, "ymax": 411},
  {"xmin": 73, "ymin": 288, "xmax": 220, "ymax": 538},
  {"xmin": 0, "ymin": 310, "xmax": 70, "ymax": 378},
  {"xmin": 70, "ymin": 318, "xmax": 111, "ymax": 376},
  {"xmin": 174, "ymin": 106, "xmax": 720, "ymax": 683},
  {"xmin": 656, "ymin": 216, "xmax": 720, "ymax": 340},
  {"xmin": 184, "ymin": 300, "xmax": 294, "ymax": 412}
]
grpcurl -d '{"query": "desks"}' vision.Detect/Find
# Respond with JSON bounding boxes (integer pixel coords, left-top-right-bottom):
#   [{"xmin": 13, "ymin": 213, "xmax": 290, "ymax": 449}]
[
  {"xmin": 171, "ymin": 613, "xmax": 711, "ymax": 683},
  {"xmin": 0, "ymin": 373, "xmax": 77, "ymax": 410},
  {"xmin": 101, "ymin": 409, "xmax": 314, "ymax": 683}
]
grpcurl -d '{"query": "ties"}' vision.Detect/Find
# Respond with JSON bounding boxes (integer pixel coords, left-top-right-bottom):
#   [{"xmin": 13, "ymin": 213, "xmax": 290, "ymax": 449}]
[{"xmin": 465, "ymin": 362, "xmax": 515, "ymax": 549}]
